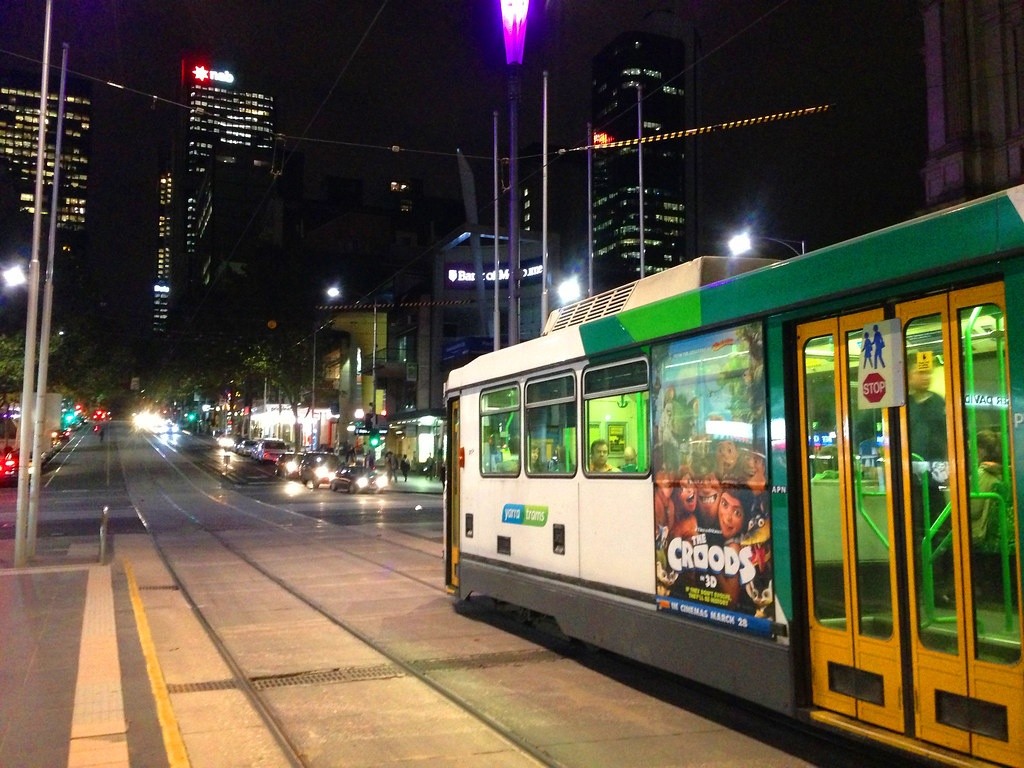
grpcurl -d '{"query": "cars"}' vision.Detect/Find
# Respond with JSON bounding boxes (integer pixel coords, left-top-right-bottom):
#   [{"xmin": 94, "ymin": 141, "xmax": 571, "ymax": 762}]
[
  {"xmin": 276, "ymin": 452, "xmax": 309, "ymax": 481},
  {"xmin": 331, "ymin": 464, "xmax": 382, "ymax": 494},
  {"xmin": 236, "ymin": 439, "xmax": 258, "ymax": 457}
]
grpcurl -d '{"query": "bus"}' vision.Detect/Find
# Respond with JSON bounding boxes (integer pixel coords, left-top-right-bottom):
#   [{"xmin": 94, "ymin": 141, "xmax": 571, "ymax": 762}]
[{"xmin": 437, "ymin": 183, "xmax": 1023, "ymax": 768}]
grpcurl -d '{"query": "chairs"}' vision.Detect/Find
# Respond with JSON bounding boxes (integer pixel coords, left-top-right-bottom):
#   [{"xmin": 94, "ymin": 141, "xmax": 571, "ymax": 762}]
[{"xmin": 936, "ymin": 478, "xmax": 1015, "ymax": 555}]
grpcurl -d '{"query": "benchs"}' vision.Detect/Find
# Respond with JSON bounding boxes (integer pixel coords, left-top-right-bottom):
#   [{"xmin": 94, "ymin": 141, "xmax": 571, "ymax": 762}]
[{"xmin": 811, "ymin": 479, "xmax": 890, "ymax": 565}]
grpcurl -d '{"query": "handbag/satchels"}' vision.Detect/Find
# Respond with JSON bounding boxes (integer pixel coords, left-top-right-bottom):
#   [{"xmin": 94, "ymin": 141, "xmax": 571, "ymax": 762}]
[{"xmin": 389, "ymin": 457, "xmax": 398, "ymax": 470}]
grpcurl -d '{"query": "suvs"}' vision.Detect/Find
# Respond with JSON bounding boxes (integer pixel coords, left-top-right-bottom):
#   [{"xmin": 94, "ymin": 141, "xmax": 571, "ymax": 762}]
[
  {"xmin": 300, "ymin": 450, "xmax": 346, "ymax": 489},
  {"xmin": 256, "ymin": 438, "xmax": 289, "ymax": 465}
]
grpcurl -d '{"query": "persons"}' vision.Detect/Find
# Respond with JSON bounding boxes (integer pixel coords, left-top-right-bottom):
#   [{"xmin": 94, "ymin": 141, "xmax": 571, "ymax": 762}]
[
  {"xmin": 812, "ymin": 443, "xmax": 844, "ymax": 483},
  {"xmin": 883, "ymin": 349, "xmax": 966, "ymax": 610},
  {"xmin": 340, "ymin": 439, "xmax": 433, "ymax": 483},
  {"xmin": 941, "ymin": 420, "xmax": 1003, "ymax": 606},
  {"xmin": 494, "ymin": 436, "xmax": 647, "ymax": 476}
]
